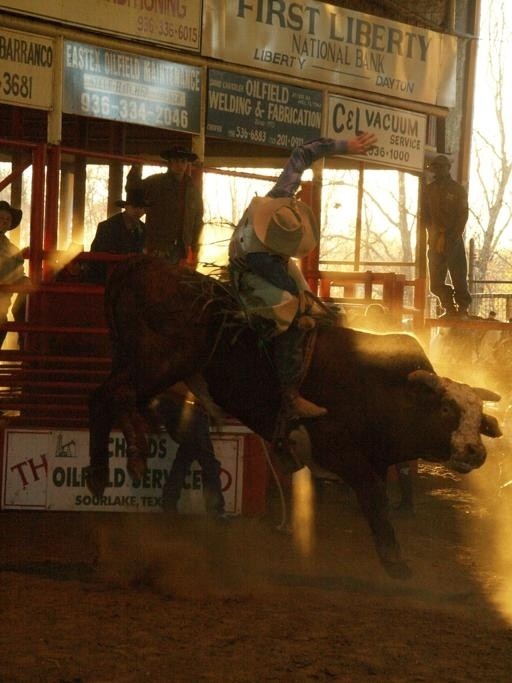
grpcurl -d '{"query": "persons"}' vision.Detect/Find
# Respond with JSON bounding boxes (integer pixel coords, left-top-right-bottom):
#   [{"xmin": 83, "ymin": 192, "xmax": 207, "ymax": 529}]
[
  {"xmin": 0, "ymin": 200, "xmax": 27, "ymax": 349},
  {"xmin": 129, "ymin": 146, "xmax": 203, "ymax": 266},
  {"xmin": 227, "ymin": 127, "xmax": 383, "ymax": 418},
  {"xmin": 418, "ymin": 155, "xmax": 474, "ymax": 320},
  {"xmin": 91, "ymin": 187, "xmax": 151, "ymax": 277}
]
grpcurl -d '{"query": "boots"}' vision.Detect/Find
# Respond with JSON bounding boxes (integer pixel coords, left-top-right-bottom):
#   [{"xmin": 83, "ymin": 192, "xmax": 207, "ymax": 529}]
[
  {"xmin": 437, "ymin": 305, "xmax": 456, "ymax": 320},
  {"xmin": 278, "ymin": 372, "xmax": 327, "ymax": 418},
  {"xmin": 457, "ymin": 310, "xmax": 469, "ymax": 320}
]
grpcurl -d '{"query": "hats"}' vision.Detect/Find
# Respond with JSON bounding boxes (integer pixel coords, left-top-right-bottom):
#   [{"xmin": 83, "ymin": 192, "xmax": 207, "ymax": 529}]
[
  {"xmin": 432, "ymin": 155, "xmax": 451, "ymax": 166},
  {"xmin": 160, "ymin": 146, "xmax": 197, "ymax": 162},
  {"xmin": 254, "ymin": 198, "xmax": 319, "ymax": 257},
  {"xmin": 0, "ymin": 201, "xmax": 22, "ymax": 229},
  {"xmin": 115, "ymin": 189, "xmax": 150, "ymax": 207}
]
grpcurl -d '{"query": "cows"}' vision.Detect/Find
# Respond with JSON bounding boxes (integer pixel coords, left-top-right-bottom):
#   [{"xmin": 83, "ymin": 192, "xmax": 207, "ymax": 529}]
[{"xmin": 85, "ymin": 255, "xmax": 504, "ymax": 581}]
